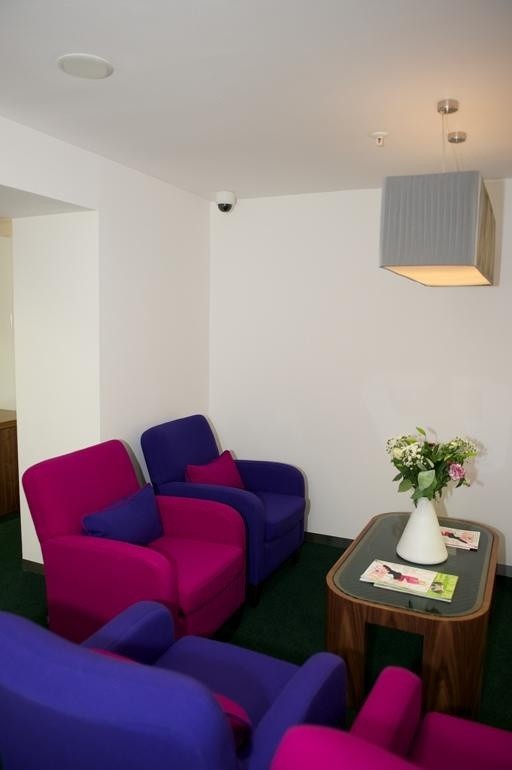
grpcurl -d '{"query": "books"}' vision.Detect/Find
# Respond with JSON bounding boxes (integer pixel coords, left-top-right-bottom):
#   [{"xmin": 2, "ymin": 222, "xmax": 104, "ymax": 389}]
[
  {"xmin": 439, "ymin": 526, "xmax": 480, "ymax": 552},
  {"xmin": 359, "ymin": 559, "xmax": 459, "ymax": 604}
]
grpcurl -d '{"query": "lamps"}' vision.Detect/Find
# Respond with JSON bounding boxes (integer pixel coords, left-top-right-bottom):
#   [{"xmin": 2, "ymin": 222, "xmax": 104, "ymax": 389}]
[{"xmin": 376, "ymin": 100, "xmax": 496, "ymax": 289}]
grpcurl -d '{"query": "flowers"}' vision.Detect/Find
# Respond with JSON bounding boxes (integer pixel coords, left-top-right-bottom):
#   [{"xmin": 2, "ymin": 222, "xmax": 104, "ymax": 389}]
[{"xmin": 385, "ymin": 425, "xmax": 480, "ymax": 509}]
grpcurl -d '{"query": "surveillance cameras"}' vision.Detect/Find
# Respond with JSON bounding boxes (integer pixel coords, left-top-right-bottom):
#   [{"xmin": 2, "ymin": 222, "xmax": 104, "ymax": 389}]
[{"xmin": 217, "ymin": 192, "xmax": 235, "ymax": 213}]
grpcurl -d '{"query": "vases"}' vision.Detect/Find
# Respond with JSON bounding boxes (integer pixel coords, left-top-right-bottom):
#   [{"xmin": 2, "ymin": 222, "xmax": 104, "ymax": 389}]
[{"xmin": 395, "ymin": 496, "xmax": 448, "ymax": 565}]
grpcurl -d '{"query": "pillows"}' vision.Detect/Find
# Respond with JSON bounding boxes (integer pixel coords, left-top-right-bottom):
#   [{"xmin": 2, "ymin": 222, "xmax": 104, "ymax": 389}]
[
  {"xmin": 186, "ymin": 450, "xmax": 244, "ymax": 490},
  {"xmin": 83, "ymin": 482, "xmax": 163, "ymax": 547},
  {"xmin": 87, "ymin": 644, "xmax": 254, "ymax": 748}
]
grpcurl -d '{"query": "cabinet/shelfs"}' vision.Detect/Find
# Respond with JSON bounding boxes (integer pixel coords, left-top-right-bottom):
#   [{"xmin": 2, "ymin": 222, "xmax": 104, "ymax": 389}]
[{"xmin": 1, "ymin": 410, "xmax": 23, "ymax": 524}]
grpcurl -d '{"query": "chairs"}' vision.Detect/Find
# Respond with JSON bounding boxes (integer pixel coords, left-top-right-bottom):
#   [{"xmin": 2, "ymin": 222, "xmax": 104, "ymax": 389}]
[
  {"xmin": 270, "ymin": 667, "xmax": 511, "ymax": 770},
  {"xmin": 24, "ymin": 438, "xmax": 248, "ymax": 648},
  {"xmin": 141, "ymin": 414, "xmax": 307, "ymax": 609},
  {"xmin": 0, "ymin": 599, "xmax": 348, "ymax": 770}
]
